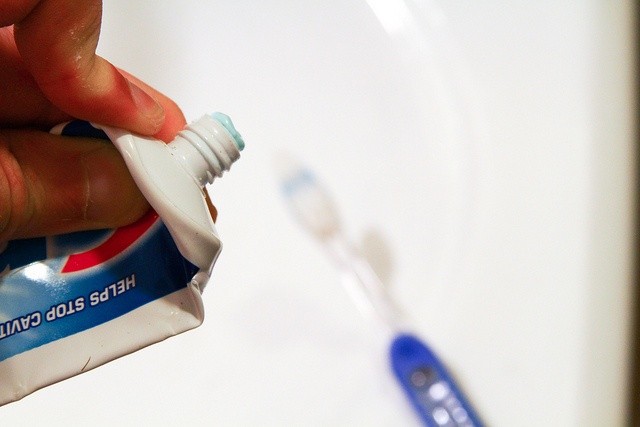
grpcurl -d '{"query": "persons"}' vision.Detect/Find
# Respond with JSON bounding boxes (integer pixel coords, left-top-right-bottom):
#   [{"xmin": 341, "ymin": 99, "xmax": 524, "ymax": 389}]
[{"xmin": 1, "ymin": 1, "xmax": 218, "ymax": 240}]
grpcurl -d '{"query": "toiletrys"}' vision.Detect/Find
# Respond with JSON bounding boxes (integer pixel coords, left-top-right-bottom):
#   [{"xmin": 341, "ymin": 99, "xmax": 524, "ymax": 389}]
[{"xmin": 1, "ymin": 112, "xmax": 247, "ymax": 407}]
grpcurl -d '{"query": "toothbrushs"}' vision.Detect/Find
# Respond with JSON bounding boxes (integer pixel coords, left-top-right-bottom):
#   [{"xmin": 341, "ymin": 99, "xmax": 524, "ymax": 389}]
[{"xmin": 278, "ymin": 169, "xmax": 488, "ymax": 427}]
